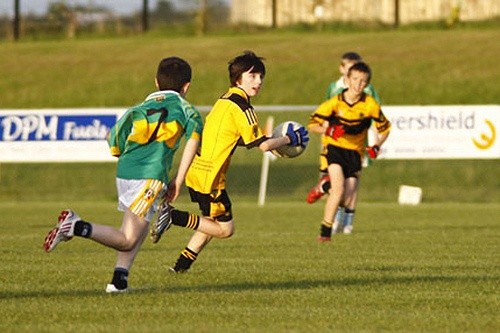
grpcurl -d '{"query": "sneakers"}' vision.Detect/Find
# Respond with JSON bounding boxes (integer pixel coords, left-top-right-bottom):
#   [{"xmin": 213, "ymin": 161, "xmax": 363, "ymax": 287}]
[
  {"xmin": 152, "ymin": 201, "xmax": 175, "ymax": 243},
  {"xmin": 167, "ymin": 266, "xmax": 187, "ymax": 275},
  {"xmin": 319, "ymin": 236, "xmax": 331, "ymax": 241},
  {"xmin": 306, "ymin": 175, "xmax": 331, "ymax": 204},
  {"xmin": 44, "ymin": 207, "xmax": 81, "ymax": 253},
  {"xmin": 106, "ymin": 283, "xmax": 128, "ymax": 293}
]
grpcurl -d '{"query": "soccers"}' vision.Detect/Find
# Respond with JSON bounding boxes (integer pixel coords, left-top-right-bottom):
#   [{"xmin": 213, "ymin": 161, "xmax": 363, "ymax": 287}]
[{"xmin": 274, "ymin": 123, "xmax": 307, "ymax": 158}]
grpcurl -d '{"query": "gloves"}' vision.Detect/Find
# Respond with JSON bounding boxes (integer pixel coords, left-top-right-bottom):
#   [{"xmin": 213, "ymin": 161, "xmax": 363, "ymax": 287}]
[
  {"xmin": 325, "ymin": 124, "xmax": 345, "ymax": 142},
  {"xmin": 365, "ymin": 145, "xmax": 380, "ymax": 159},
  {"xmin": 285, "ymin": 123, "xmax": 309, "ymax": 148}
]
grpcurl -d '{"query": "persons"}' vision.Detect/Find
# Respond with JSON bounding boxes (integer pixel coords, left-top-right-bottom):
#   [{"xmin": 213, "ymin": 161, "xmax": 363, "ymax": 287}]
[
  {"xmin": 43, "ymin": 56, "xmax": 203, "ymax": 293},
  {"xmin": 325, "ymin": 51, "xmax": 380, "ymax": 235},
  {"xmin": 305, "ymin": 61, "xmax": 392, "ymax": 242},
  {"xmin": 150, "ymin": 50, "xmax": 310, "ymax": 274}
]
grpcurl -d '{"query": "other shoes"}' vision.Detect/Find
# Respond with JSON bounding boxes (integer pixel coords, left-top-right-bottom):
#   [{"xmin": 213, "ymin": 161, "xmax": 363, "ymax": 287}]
[
  {"xmin": 333, "ymin": 223, "xmax": 340, "ymax": 233},
  {"xmin": 343, "ymin": 226, "xmax": 352, "ymax": 234}
]
